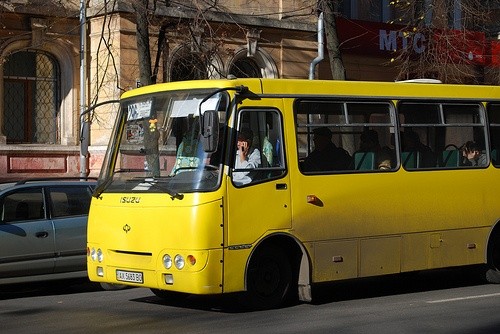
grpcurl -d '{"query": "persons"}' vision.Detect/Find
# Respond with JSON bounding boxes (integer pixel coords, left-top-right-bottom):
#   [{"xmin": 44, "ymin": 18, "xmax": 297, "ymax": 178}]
[
  {"xmin": 201, "ymin": 126, "xmax": 262, "ymax": 187},
  {"xmin": 353, "ymin": 128, "xmax": 400, "ymax": 171},
  {"xmin": 461, "ymin": 141, "xmax": 488, "ymax": 168},
  {"xmin": 300, "ymin": 127, "xmax": 357, "ymax": 175},
  {"xmin": 400, "ymin": 126, "xmax": 440, "ymax": 171}
]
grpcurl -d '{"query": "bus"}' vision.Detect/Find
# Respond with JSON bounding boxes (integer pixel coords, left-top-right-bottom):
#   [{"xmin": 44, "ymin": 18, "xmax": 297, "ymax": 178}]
[{"xmin": 79, "ymin": 78, "xmax": 500, "ymax": 311}]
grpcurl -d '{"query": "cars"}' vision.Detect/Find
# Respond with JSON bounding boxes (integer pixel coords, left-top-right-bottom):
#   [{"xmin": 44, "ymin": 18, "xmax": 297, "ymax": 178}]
[{"xmin": 0, "ymin": 176, "xmax": 130, "ymax": 291}]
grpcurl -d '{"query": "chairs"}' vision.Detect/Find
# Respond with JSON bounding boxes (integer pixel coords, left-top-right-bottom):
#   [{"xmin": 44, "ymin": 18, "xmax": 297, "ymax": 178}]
[
  {"xmin": 253, "ymin": 133, "xmax": 273, "ymax": 177},
  {"xmin": 15, "ymin": 201, "xmax": 30, "ymax": 221},
  {"xmin": 170, "ymin": 133, "xmax": 206, "ymax": 176},
  {"xmin": 354, "ymin": 149, "xmax": 500, "ymax": 171}
]
